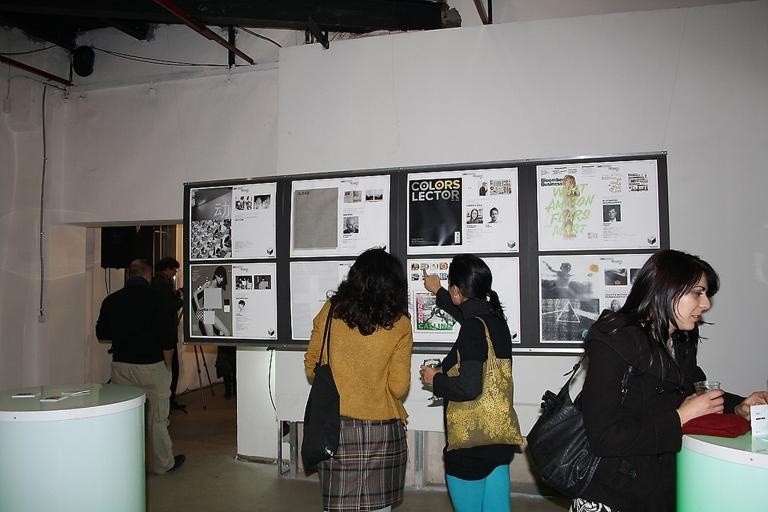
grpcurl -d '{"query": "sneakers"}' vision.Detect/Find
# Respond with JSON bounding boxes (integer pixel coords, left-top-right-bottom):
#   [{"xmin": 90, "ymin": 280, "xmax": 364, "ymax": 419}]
[{"xmin": 166, "ymin": 454, "xmax": 185, "ymax": 474}]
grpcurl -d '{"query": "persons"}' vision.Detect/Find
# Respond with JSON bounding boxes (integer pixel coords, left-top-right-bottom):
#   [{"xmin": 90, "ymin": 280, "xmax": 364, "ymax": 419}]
[
  {"xmin": 304, "ymin": 246, "xmax": 413, "ymax": 512},
  {"xmin": 560, "ymin": 175, "xmax": 580, "ymax": 238},
  {"xmin": 479, "ymin": 181, "xmax": 488, "ymax": 196},
  {"xmin": 487, "ymin": 207, "xmax": 502, "ymax": 223},
  {"xmin": 258, "ymin": 276, "xmax": 271, "ymax": 289},
  {"xmin": 610, "ymin": 299, "xmax": 622, "ymax": 313},
  {"xmin": 604, "ymin": 205, "xmax": 621, "ymax": 222},
  {"xmin": 546, "ymin": 261, "xmax": 575, "ymax": 298},
  {"xmin": 343, "ymin": 217, "xmax": 360, "ymax": 233},
  {"xmin": 211, "ymin": 343, "xmax": 236, "ymax": 400},
  {"xmin": 237, "ymin": 299, "xmax": 246, "ymax": 315},
  {"xmin": 565, "ymin": 247, "xmax": 768, "ymax": 511},
  {"xmin": 151, "ymin": 256, "xmax": 189, "ymax": 410},
  {"xmin": 95, "ymin": 259, "xmax": 184, "ymax": 475},
  {"xmin": 468, "ymin": 208, "xmax": 484, "ymax": 224},
  {"xmin": 193, "ymin": 266, "xmax": 231, "ymax": 336},
  {"xmin": 418, "ymin": 256, "xmax": 525, "ymax": 510}
]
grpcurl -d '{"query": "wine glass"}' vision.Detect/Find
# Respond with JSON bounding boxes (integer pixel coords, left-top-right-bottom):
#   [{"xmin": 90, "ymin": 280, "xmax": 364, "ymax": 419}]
[{"xmin": 423, "ymin": 359, "xmax": 442, "ymax": 401}]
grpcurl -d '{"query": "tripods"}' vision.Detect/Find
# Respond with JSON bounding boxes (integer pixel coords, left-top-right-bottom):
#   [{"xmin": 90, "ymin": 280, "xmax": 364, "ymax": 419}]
[{"xmin": 176, "ymin": 308, "xmax": 216, "ymax": 410}]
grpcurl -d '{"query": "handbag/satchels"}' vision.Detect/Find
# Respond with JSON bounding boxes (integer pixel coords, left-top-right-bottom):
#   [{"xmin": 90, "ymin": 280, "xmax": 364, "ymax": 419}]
[
  {"xmin": 444, "ymin": 316, "xmax": 524, "ymax": 454},
  {"xmin": 301, "ymin": 295, "xmax": 341, "ymax": 477},
  {"xmin": 526, "ymin": 347, "xmax": 632, "ymax": 510}
]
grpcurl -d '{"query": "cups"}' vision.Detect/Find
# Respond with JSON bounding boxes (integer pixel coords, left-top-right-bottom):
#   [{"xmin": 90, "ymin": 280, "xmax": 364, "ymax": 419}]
[{"xmin": 695, "ymin": 380, "xmax": 721, "ymax": 397}]
